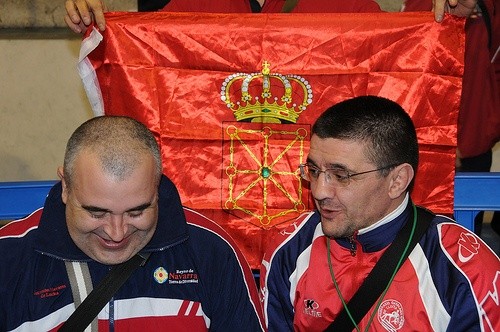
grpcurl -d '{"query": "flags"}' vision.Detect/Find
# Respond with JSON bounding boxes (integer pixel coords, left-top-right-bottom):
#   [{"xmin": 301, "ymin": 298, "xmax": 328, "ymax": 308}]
[{"xmin": 77, "ymin": 11, "xmax": 467, "ymax": 268}]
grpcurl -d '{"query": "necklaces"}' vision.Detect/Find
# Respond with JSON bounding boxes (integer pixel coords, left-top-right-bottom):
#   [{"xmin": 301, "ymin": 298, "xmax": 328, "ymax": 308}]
[{"xmin": 326, "ymin": 205, "xmax": 418, "ymax": 332}]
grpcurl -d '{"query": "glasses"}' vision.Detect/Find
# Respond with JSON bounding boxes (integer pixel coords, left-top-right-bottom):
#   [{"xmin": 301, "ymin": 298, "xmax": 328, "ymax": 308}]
[{"xmin": 298, "ymin": 161, "xmax": 411, "ymax": 188}]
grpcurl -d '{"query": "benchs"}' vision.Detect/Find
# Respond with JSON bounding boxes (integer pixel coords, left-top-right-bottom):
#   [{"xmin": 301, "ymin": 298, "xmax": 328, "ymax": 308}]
[{"xmin": 0, "ymin": 171, "xmax": 500, "ymax": 234}]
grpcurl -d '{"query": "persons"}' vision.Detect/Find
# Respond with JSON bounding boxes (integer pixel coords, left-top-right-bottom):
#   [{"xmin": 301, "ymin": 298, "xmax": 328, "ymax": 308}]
[
  {"xmin": 259, "ymin": 94, "xmax": 500, "ymax": 332},
  {"xmin": 0, "ymin": 115, "xmax": 259, "ymax": 332},
  {"xmin": 62, "ymin": 0, "xmax": 500, "ymax": 234}
]
